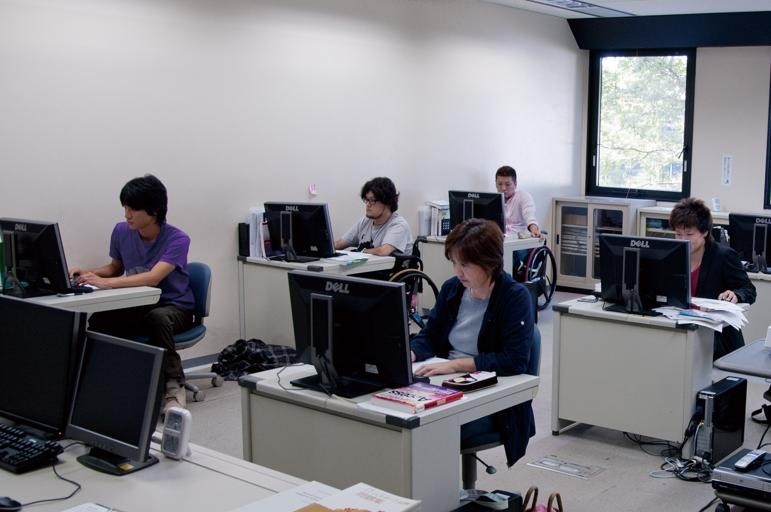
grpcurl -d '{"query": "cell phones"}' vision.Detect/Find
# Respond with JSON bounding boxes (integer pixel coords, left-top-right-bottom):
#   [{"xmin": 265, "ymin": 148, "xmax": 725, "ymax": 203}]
[{"xmin": 733, "ymin": 450, "xmax": 767, "ymax": 471}]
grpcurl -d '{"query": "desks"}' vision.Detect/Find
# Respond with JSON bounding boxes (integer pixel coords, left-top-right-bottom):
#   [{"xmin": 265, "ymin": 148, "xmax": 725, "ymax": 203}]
[
  {"xmin": 553, "ymin": 294, "xmax": 714, "ymax": 461},
  {"xmin": 713, "ymin": 338, "xmax": 770, "ymax": 378},
  {"xmin": 238, "ymin": 356, "xmax": 540, "ymax": 511},
  {"xmin": 237, "ymin": 249, "xmax": 396, "ymax": 351},
  {"xmin": 0, "ymin": 428, "xmax": 311, "ymax": 512},
  {"xmin": 419, "ymin": 233, "xmax": 545, "ymax": 316},
  {"xmin": 23, "ymin": 283, "xmax": 162, "ymax": 333},
  {"xmin": 741, "ymin": 271, "xmax": 770, "ymax": 345}
]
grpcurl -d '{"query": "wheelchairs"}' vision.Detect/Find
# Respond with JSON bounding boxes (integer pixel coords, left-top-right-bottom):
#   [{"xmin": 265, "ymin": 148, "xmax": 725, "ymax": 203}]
[
  {"xmin": 390, "ymin": 235, "xmax": 440, "ymax": 336},
  {"xmin": 513, "ymin": 232, "xmax": 557, "ymax": 310}
]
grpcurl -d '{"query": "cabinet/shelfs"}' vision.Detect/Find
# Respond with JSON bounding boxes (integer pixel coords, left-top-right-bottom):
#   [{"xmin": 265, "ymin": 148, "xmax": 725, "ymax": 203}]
[
  {"xmin": 553, "ymin": 195, "xmax": 657, "ymax": 291},
  {"xmin": 637, "ymin": 206, "xmax": 730, "ymax": 236}
]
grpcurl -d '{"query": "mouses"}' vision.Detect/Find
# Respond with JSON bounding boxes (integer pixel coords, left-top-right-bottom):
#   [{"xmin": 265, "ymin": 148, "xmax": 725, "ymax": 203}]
[{"xmin": 0, "ymin": 498, "xmax": 21, "ymax": 512}]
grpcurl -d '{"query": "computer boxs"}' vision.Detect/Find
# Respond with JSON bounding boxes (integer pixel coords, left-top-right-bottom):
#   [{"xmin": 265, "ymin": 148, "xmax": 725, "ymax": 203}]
[
  {"xmin": 693, "ymin": 376, "xmax": 747, "ymax": 465},
  {"xmin": 752, "ymin": 219, "xmax": 770, "ymax": 268}
]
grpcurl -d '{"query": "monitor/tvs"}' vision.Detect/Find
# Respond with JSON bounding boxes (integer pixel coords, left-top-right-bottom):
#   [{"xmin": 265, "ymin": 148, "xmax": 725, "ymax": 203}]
[
  {"xmin": 448, "ymin": 190, "xmax": 506, "ymax": 234},
  {"xmin": 728, "ymin": 213, "xmax": 771, "ymax": 274},
  {"xmin": 598, "ymin": 233, "xmax": 691, "ymax": 317},
  {"xmin": 64, "ymin": 330, "xmax": 168, "ymax": 477},
  {"xmin": 286, "ymin": 269, "xmax": 413, "ymax": 400},
  {"xmin": 0, "ymin": 294, "xmax": 87, "ymax": 442},
  {"xmin": 263, "ymin": 202, "xmax": 335, "ymax": 263},
  {"xmin": 0, "ymin": 220, "xmax": 72, "ymax": 298}
]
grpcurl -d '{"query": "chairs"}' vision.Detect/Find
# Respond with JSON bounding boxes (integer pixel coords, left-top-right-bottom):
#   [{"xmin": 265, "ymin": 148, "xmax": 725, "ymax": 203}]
[
  {"xmin": 460, "ymin": 321, "xmax": 541, "ymax": 489},
  {"xmin": 136, "ymin": 261, "xmax": 224, "ymax": 402}
]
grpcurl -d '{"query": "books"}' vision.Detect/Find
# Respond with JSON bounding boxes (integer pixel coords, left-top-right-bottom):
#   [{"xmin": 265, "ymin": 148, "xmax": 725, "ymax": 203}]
[
  {"xmin": 291, "ymin": 482, "xmax": 422, "ymax": 512},
  {"xmin": 651, "ymin": 297, "xmax": 748, "ymax": 335},
  {"xmin": 321, "ymin": 256, "xmax": 368, "ymax": 268},
  {"xmin": 248, "ymin": 207, "xmax": 273, "ymax": 258},
  {"xmin": 373, "ymin": 381, "xmax": 463, "ymax": 417},
  {"xmin": 419, "ymin": 200, "xmax": 450, "ymax": 238}
]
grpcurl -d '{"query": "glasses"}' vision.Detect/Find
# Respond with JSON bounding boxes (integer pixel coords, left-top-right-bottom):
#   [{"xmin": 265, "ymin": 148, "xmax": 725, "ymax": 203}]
[{"xmin": 363, "ymin": 199, "xmax": 378, "ymax": 206}]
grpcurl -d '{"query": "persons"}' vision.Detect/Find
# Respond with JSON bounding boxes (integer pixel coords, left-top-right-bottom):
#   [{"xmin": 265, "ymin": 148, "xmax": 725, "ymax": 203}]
[
  {"xmin": 492, "ymin": 166, "xmax": 545, "ymax": 241},
  {"xmin": 669, "ymin": 198, "xmax": 756, "ymax": 361},
  {"xmin": 333, "ymin": 175, "xmax": 413, "ymax": 283},
  {"xmin": 408, "ymin": 219, "xmax": 537, "ymax": 468},
  {"xmin": 69, "ymin": 173, "xmax": 197, "ymax": 422}
]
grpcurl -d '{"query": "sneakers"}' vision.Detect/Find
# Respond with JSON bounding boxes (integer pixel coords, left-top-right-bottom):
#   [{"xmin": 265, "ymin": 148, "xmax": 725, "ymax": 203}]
[{"xmin": 159, "ymin": 385, "xmax": 187, "ymax": 418}]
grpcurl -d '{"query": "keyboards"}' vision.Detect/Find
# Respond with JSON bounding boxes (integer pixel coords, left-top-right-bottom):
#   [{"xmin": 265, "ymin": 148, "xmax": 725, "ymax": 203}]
[
  {"xmin": 0, "ymin": 422, "xmax": 63, "ymax": 475},
  {"xmin": 412, "ymin": 373, "xmax": 430, "ymax": 385},
  {"xmin": 70, "ymin": 285, "xmax": 93, "ymax": 295},
  {"xmin": 332, "ymin": 252, "xmax": 348, "ymax": 258}
]
